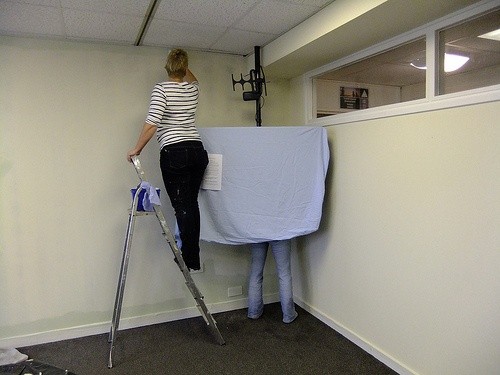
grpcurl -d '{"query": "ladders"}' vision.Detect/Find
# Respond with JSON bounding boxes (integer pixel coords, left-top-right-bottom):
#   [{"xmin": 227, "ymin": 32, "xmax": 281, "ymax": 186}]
[{"xmin": 105, "ymin": 153, "xmax": 226, "ymax": 368}]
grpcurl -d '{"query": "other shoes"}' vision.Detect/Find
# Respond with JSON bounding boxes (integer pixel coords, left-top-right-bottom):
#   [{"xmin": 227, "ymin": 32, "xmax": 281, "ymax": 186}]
[{"xmin": 174, "ymin": 258, "xmax": 201, "ymax": 273}]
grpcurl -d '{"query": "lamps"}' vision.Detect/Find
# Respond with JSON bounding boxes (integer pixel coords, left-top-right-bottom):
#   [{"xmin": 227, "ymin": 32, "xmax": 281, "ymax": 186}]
[{"xmin": 442, "ymin": 52, "xmax": 472, "ymax": 73}]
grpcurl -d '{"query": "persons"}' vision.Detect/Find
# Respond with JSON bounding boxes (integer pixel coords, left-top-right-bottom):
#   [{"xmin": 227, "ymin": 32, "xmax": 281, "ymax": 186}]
[
  {"xmin": 246, "ymin": 239, "xmax": 299, "ymax": 323},
  {"xmin": 126, "ymin": 47, "xmax": 211, "ymax": 275}
]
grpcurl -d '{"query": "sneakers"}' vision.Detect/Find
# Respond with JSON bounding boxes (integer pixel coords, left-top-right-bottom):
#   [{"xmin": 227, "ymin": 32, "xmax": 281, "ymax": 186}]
[
  {"xmin": 282, "ymin": 310, "xmax": 299, "ymax": 323},
  {"xmin": 247, "ymin": 310, "xmax": 263, "ymax": 319}
]
